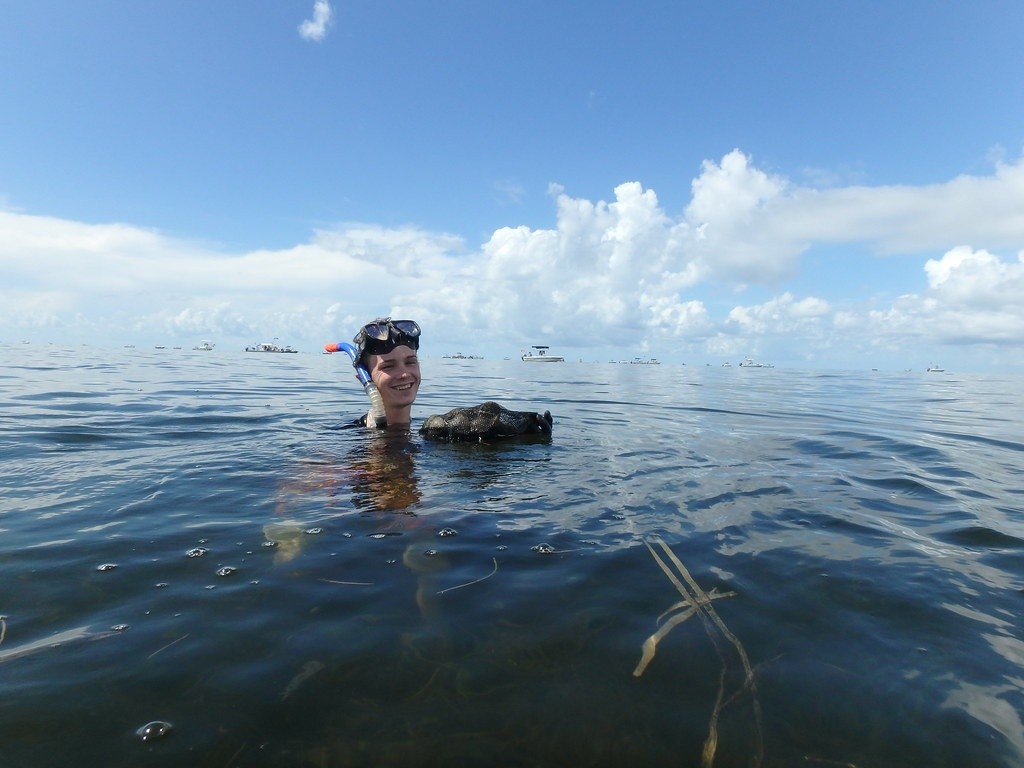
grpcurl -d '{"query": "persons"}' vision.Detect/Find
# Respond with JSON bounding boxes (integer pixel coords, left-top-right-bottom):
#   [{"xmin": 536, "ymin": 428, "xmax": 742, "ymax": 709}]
[{"xmin": 352, "ymin": 317, "xmax": 422, "ymax": 424}]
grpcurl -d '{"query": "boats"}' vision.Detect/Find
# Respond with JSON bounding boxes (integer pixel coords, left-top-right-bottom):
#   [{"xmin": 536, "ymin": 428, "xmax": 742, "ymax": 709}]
[
  {"xmin": 441, "ymin": 355, "xmax": 485, "ymax": 359},
  {"xmin": 607, "ymin": 357, "xmax": 661, "ymax": 364},
  {"xmin": 927, "ymin": 367, "xmax": 945, "ymax": 372},
  {"xmin": 243, "ymin": 344, "xmax": 299, "ymax": 353},
  {"xmin": 521, "ymin": 345, "xmax": 563, "ymax": 362},
  {"xmin": 722, "ymin": 357, "xmax": 774, "ymax": 368},
  {"xmin": 173, "ymin": 347, "xmax": 181, "ymax": 350},
  {"xmin": 155, "ymin": 345, "xmax": 165, "ymax": 349},
  {"xmin": 192, "ymin": 340, "xmax": 215, "ymax": 351}
]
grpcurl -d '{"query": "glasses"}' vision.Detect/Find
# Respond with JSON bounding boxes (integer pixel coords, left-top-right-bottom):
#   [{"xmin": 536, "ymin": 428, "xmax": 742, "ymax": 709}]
[{"xmin": 354, "ymin": 320, "xmax": 422, "ymax": 356}]
